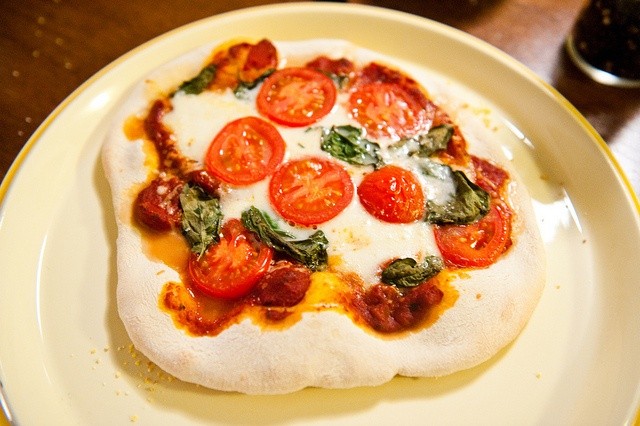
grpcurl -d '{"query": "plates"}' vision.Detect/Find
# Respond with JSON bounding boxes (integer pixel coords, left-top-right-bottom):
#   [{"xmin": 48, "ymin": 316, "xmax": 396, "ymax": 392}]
[{"xmin": 1, "ymin": 2, "xmax": 640, "ymax": 426}]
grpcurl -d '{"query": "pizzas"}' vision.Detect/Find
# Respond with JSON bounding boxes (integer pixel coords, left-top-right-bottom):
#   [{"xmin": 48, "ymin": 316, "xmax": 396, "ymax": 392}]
[{"xmin": 99, "ymin": 34, "xmax": 543, "ymax": 394}]
